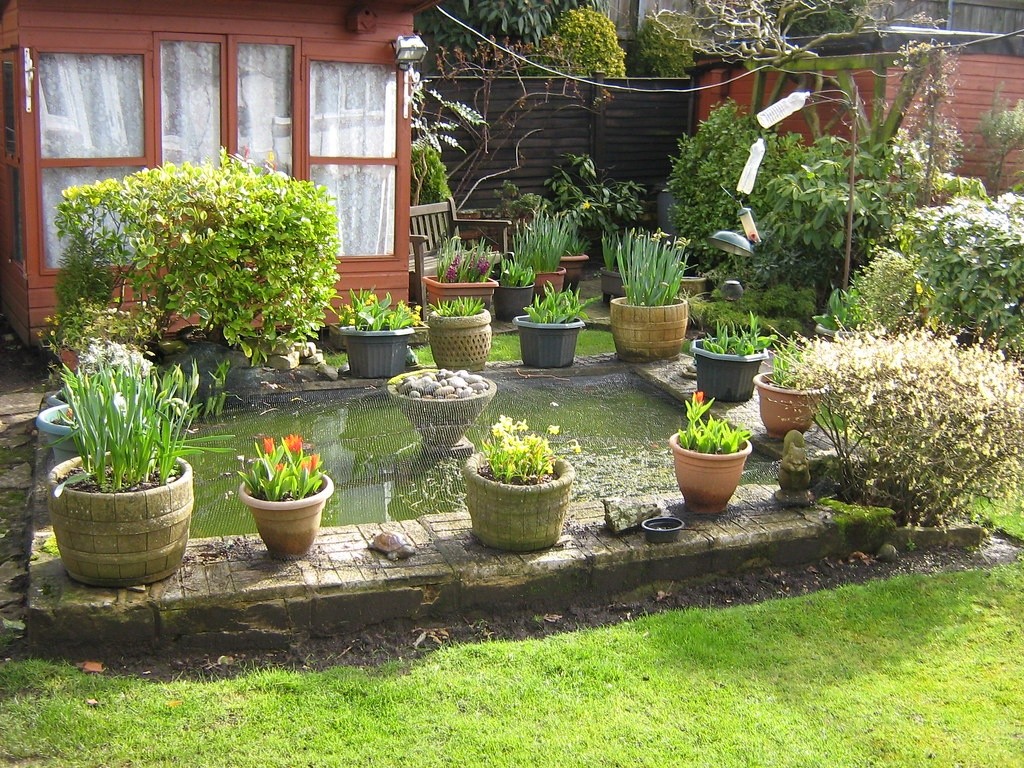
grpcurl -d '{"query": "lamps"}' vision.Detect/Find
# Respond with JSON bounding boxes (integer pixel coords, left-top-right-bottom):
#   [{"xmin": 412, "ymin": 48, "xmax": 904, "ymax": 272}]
[{"xmin": 394, "ymin": 34, "xmax": 429, "ymax": 71}]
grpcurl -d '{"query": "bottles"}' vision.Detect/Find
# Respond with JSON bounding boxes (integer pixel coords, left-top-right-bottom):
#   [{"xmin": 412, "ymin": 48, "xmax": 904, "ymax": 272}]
[
  {"xmin": 736, "ymin": 138, "xmax": 765, "ymax": 195},
  {"xmin": 756, "ymin": 91, "xmax": 810, "ymax": 129}
]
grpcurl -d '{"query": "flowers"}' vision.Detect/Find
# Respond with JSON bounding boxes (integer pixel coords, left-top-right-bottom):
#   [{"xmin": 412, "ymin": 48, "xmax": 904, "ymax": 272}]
[
  {"xmin": 37, "ymin": 295, "xmax": 162, "ymax": 351},
  {"xmin": 349, "ymin": 285, "xmax": 420, "ymax": 331},
  {"xmin": 237, "ymin": 433, "xmax": 328, "ymax": 502},
  {"xmin": 436, "ymin": 236, "xmax": 496, "ymax": 283},
  {"xmin": 67, "ymin": 340, "xmax": 159, "ymax": 430},
  {"xmin": 480, "ymin": 414, "xmax": 583, "ymax": 484},
  {"xmin": 338, "ymin": 301, "xmax": 422, "ymax": 327},
  {"xmin": 678, "ymin": 391, "xmax": 754, "ymax": 455}
]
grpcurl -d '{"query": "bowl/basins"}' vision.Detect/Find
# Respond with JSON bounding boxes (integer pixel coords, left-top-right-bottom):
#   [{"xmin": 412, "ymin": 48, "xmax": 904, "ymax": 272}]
[
  {"xmin": 707, "ymin": 231, "xmax": 755, "ymax": 258},
  {"xmin": 642, "ymin": 516, "xmax": 684, "ymax": 543}
]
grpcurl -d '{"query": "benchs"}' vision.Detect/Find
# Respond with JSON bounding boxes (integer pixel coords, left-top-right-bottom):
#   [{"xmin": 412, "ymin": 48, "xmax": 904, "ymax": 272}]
[{"xmin": 409, "ymin": 197, "xmax": 512, "ymax": 306}]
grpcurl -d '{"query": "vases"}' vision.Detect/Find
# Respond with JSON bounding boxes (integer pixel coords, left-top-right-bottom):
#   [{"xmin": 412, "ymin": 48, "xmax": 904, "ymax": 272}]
[
  {"xmin": 56, "ymin": 344, "xmax": 81, "ymax": 377},
  {"xmin": 339, "ymin": 326, "xmax": 415, "ymax": 379},
  {"xmin": 641, "ymin": 516, "xmax": 684, "ymax": 543},
  {"xmin": 329, "ymin": 322, "xmax": 429, "ymax": 350},
  {"xmin": 670, "ymin": 430, "xmax": 752, "ymax": 514},
  {"xmin": 238, "ymin": 472, "xmax": 334, "ymax": 559},
  {"xmin": 36, "ymin": 403, "xmax": 162, "ymax": 466},
  {"xmin": 423, "ymin": 275, "xmax": 500, "ymax": 312},
  {"xmin": 48, "ymin": 389, "xmax": 69, "ymax": 408},
  {"xmin": 464, "ymin": 451, "xmax": 575, "ymax": 551}
]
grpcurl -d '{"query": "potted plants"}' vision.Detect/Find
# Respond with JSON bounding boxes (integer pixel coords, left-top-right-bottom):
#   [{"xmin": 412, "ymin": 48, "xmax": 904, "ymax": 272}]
[
  {"xmin": 46, "ymin": 356, "xmax": 207, "ymax": 589},
  {"xmin": 690, "ymin": 312, "xmax": 778, "ymax": 403},
  {"xmin": 812, "ymin": 286, "xmax": 887, "ymax": 343},
  {"xmin": 609, "ymin": 227, "xmax": 692, "ymax": 362},
  {"xmin": 751, "ymin": 336, "xmax": 828, "ymax": 440},
  {"xmin": 558, "ymin": 231, "xmax": 592, "ymax": 294},
  {"xmin": 426, "ymin": 296, "xmax": 492, "ymax": 372},
  {"xmin": 494, "ymin": 255, "xmax": 536, "ymax": 321},
  {"xmin": 512, "ymin": 201, "xmax": 575, "ymax": 309},
  {"xmin": 511, "ymin": 280, "xmax": 602, "ymax": 368}
]
